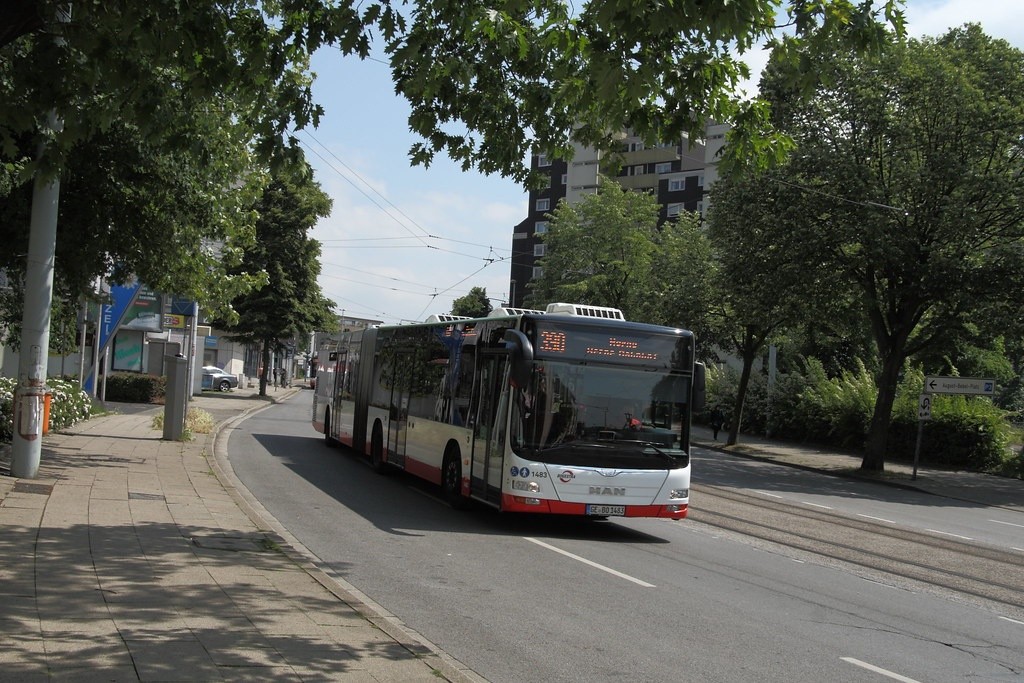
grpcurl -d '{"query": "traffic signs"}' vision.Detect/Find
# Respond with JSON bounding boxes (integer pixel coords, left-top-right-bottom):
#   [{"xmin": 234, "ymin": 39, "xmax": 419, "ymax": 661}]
[
  {"xmin": 924, "ymin": 374, "xmax": 997, "ymax": 397},
  {"xmin": 918, "ymin": 393, "xmax": 933, "ymax": 421}
]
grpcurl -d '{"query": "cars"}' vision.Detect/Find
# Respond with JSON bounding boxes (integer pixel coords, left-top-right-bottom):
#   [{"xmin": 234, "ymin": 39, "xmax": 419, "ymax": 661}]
[{"xmin": 202, "ymin": 365, "xmax": 239, "ymax": 392}]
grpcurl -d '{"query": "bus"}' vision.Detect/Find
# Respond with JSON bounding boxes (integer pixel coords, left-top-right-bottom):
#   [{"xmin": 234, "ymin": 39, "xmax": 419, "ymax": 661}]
[{"xmin": 309, "ymin": 302, "xmax": 708, "ymax": 519}]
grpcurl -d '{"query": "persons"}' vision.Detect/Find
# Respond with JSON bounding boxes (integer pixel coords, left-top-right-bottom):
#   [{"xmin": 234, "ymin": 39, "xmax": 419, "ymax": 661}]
[{"xmin": 711, "ymin": 405, "xmax": 723, "ymax": 441}]
